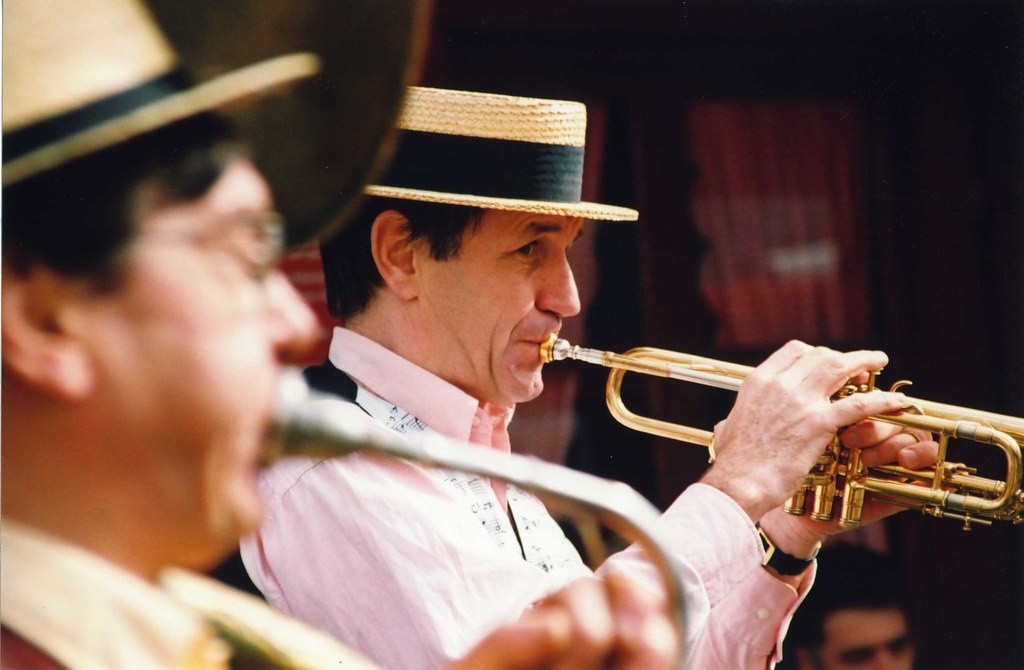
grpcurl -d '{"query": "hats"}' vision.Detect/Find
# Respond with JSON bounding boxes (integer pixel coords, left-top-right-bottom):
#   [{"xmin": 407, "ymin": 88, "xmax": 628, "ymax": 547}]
[
  {"xmin": 2, "ymin": 0, "xmax": 320, "ymax": 187},
  {"xmin": 364, "ymin": 84, "xmax": 641, "ymax": 223}
]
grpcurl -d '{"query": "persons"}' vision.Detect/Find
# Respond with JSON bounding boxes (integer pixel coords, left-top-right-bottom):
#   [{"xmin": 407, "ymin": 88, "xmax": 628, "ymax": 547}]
[
  {"xmin": 238, "ymin": 86, "xmax": 969, "ymax": 670},
  {"xmin": 0, "ymin": 0, "xmax": 680, "ymax": 670}
]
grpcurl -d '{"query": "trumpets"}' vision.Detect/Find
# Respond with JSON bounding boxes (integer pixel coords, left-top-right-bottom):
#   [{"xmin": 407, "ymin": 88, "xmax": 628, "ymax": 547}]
[
  {"xmin": 538, "ymin": 331, "xmax": 1024, "ymax": 532},
  {"xmin": 252, "ymin": 370, "xmax": 700, "ymax": 669}
]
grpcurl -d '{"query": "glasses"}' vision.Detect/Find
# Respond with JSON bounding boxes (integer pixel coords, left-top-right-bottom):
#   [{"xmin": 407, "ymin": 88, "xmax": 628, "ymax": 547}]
[{"xmin": 33, "ymin": 213, "xmax": 283, "ymax": 276}]
